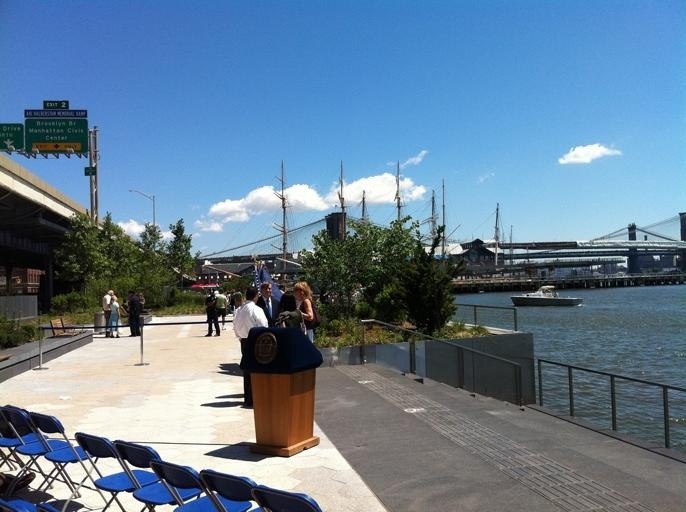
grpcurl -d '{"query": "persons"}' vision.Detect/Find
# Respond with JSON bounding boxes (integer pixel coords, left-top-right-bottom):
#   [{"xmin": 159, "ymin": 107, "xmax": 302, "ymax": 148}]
[
  {"xmin": 205, "ymin": 279, "xmax": 322, "ymax": 409},
  {"xmin": 101, "ymin": 288, "xmax": 145, "ymax": 338}
]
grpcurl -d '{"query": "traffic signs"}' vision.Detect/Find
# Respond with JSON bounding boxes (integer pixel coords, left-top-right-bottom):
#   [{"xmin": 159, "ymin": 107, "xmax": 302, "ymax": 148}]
[
  {"xmin": 0, "ymin": 123, "xmax": 23, "ymax": 150},
  {"xmin": 22, "ymin": 107, "xmax": 87, "ymax": 119},
  {"xmin": 26, "ymin": 119, "xmax": 87, "ymax": 153}
]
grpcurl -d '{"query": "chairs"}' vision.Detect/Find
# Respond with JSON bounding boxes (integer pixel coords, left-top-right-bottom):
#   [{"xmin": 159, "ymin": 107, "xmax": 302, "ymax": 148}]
[
  {"xmin": 198, "ymin": 470, "xmax": 264, "ymax": 511},
  {"xmin": 0, "ymin": 404, "xmax": 160, "ymax": 512},
  {"xmin": 150, "ymin": 459, "xmax": 253, "ymax": 511},
  {"xmin": 114, "ymin": 439, "xmax": 202, "ymax": 511},
  {"xmin": 252, "ymin": 483, "xmax": 321, "ymax": 511}
]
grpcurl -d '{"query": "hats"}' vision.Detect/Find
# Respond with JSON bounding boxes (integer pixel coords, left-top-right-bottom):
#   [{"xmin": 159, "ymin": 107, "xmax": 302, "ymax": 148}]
[{"xmin": 107, "ymin": 289, "xmax": 114, "ymax": 296}]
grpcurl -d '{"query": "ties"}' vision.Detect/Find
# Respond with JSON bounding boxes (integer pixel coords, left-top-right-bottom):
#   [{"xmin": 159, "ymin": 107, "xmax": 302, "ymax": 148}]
[{"xmin": 266, "ymin": 299, "xmax": 272, "ymax": 316}]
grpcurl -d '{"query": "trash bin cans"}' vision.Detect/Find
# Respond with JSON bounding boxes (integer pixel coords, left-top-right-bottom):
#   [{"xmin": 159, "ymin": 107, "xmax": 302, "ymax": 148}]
[{"xmin": 94, "ymin": 312, "xmax": 106, "ymax": 334}]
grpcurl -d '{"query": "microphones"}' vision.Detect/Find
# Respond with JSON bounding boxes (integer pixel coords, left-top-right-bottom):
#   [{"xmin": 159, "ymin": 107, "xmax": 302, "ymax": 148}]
[
  {"xmin": 276, "ymin": 314, "xmax": 286, "ymax": 321},
  {"xmin": 282, "ymin": 314, "xmax": 292, "ymax": 320}
]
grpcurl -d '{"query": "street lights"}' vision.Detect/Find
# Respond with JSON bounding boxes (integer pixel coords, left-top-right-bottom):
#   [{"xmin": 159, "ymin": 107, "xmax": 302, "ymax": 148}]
[{"xmin": 128, "ymin": 188, "xmax": 156, "ymax": 253}]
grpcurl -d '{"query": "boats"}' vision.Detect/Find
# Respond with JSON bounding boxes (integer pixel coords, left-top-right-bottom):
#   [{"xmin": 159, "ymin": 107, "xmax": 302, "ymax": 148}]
[{"xmin": 510, "ymin": 284, "xmax": 584, "ymax": 308}]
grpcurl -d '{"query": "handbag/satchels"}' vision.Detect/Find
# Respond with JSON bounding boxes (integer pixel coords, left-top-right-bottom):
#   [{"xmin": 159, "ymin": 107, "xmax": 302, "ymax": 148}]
[{"xmin": 303, "ymin": 302, "xmax": 322, "ymax": 330}]
